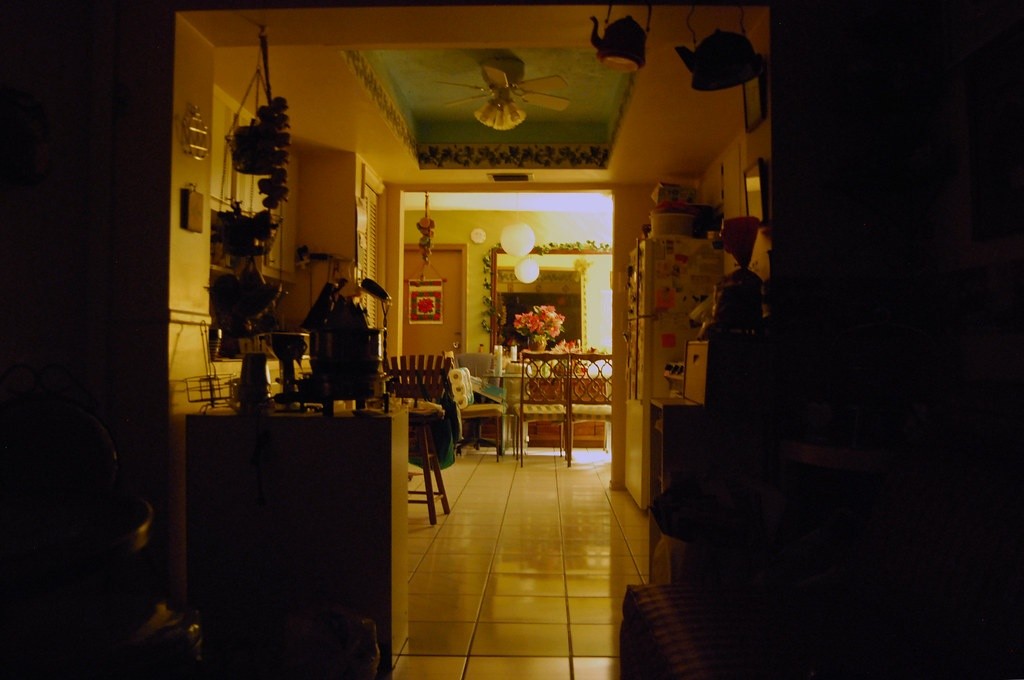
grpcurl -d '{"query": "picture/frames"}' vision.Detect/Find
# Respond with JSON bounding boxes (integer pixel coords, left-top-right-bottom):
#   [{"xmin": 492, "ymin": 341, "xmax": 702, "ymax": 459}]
[
  {"xmin": 744, "ymin": 157, "xmax": 768, "ymax": 225},
  {"xmin": 742, "ymin": 59, "xmax": 768, "ymax": 134}
]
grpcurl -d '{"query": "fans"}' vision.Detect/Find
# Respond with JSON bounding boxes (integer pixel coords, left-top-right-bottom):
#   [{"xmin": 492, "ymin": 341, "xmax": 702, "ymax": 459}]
[{"xmin": 436, "ymin": 57, "xmax": 571, "ymax": 112}]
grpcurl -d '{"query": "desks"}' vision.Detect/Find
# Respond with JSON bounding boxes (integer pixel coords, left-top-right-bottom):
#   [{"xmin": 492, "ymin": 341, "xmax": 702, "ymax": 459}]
[{"xmin": 186, "ymin": 407, "xmax": 410, "ymax": 674}]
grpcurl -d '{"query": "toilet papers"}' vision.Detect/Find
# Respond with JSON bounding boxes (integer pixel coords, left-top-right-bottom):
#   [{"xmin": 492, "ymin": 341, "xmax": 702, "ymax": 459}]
[{"xmin": 448, "ymin": 366, "xmax": 475, "ymax": 410}]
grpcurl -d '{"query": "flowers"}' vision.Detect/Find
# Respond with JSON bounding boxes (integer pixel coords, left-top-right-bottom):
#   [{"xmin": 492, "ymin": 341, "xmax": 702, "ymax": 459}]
[{"xmin": 513, "ymin": 305, "xmax": 567, "ymax": 346}]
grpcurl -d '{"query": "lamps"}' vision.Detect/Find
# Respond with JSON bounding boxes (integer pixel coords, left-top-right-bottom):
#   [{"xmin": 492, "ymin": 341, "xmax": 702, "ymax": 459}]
[
  {"xmin": 501, "ymin": 193, "xmax": 535, "ymax": 257},
  {"xmin": 514, "ymin": 255, "xmax": 539, "ymax": 283},
  {"xmin": 473, "ymin": 99, "xmax": 529, "ymax": 131}
]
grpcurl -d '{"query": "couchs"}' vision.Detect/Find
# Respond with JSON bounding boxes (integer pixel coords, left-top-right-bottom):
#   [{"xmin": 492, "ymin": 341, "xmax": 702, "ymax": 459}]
[{"xmin": 619, "ymin": 451, "xmax": 1024, "ymax": 680}]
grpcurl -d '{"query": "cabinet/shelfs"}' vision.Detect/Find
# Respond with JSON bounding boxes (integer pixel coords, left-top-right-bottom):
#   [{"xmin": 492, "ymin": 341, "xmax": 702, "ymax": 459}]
[
  {"xmin": 211, "ymin": 84, "xmax": 274, "ymax": 221},
  {"xmin": 648, "ymin": 397, "xmax": 710, "ymax": 584}
]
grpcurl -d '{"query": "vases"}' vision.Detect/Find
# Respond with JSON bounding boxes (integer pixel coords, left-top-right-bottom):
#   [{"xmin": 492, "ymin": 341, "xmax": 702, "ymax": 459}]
[{"xmin": 528, "ymin": 335, "xmax": 546, "ymax": 351}]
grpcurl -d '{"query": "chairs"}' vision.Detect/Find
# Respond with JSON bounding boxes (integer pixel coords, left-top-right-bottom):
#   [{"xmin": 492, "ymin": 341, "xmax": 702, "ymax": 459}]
[
  {"xmin": 512, "ymin": 352, "xmax": 571, "ymax": 468},
  {"xmin": 442, "ymin": 350, "xmax": 503, "ymax": 463},
  {"xmin": 382, "ymin": 354, "xmax": 452, "ymax": 526},
  {"xmin": 568, "ymin": 353, "xmax": 612, "ymax": 467}
]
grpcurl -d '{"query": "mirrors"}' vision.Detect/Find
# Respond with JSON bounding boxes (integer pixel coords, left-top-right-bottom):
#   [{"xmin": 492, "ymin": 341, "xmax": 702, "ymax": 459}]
[{"xmin": 490, "ymin": 247, "xmax": 613, "ymax": 373}]
